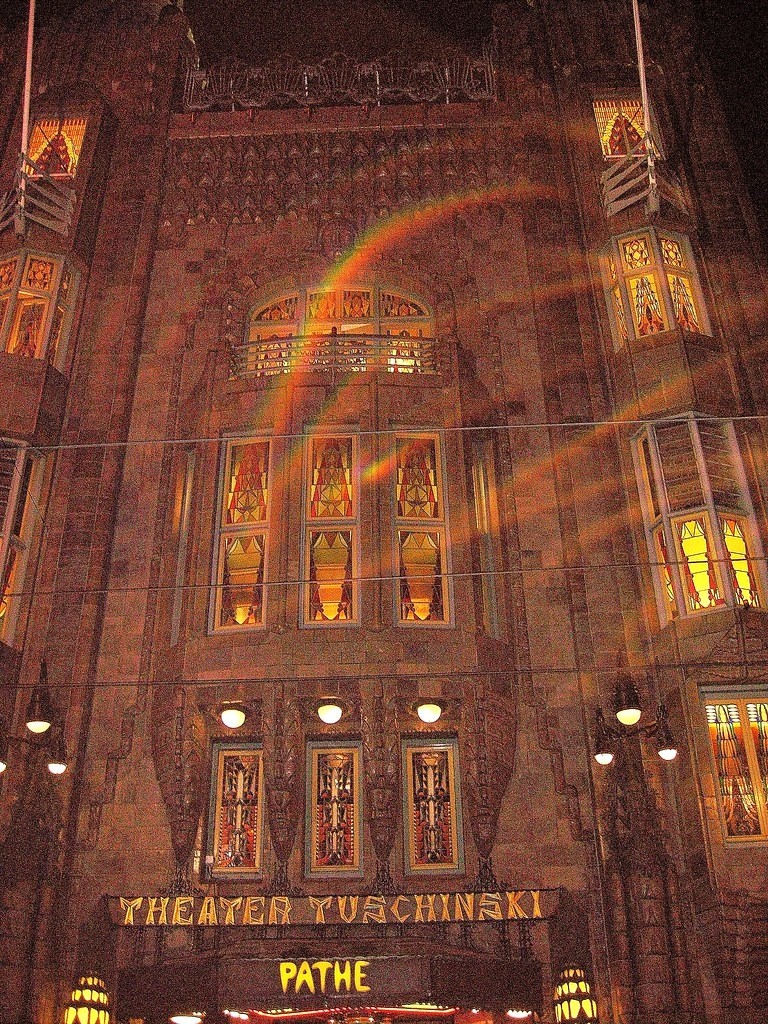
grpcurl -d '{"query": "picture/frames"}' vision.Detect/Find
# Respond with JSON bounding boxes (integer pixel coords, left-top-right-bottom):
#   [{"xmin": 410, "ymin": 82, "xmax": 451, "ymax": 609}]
[
  {"xmin": 402, "ymin": 740, "xmax": 466, "ymax": 876},
  {"xmin": 304, "ymin": 740, "xmax": 364, "ymax": 880},
  {"xmin": 203, "ymin": 743, "xmax": 263, "ymax": 882}
]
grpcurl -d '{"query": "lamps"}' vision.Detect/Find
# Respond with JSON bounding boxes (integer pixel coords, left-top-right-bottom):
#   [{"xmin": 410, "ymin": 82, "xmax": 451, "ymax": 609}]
[
  {"xmin": 413, "ymin": 699, "xmax": 449, "ymax": 722},
  {"xmin": 593, "ymin": 708, "xmax": 614, "ymax": 765},
  {"xmin": 655, "ymin": 708, "xmax": 677, "ymax": 760},
  {"xmin": 315, "ymin": 699, "xmax": 346, "ymax": 724},
  {"xmin": 46, "ymin": 708, "xmax": 67, "ymax": 775},
  {"xmin": 219, "ymin": 703, "xmax": 246, "ymax": 728},
  {"xmin": 25, "ymin": 662, "xmax": 54, "ymax": 733},
  {"xmin": 0, "ymin": 721, "xmax": 8, "ymax": 772},
  {"xmin": 615, "ymin": 683, "xmax": 642, "ymax": 725}
]
